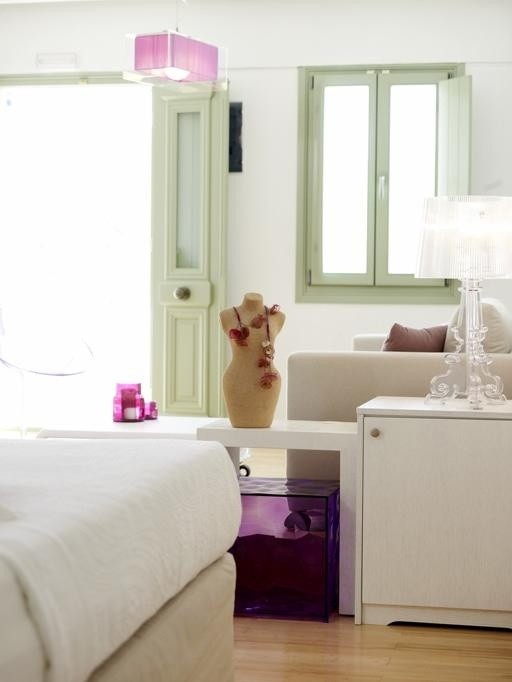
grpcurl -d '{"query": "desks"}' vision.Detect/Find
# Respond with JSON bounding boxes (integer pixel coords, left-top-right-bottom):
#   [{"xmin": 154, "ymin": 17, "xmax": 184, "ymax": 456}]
[
  {"xmin": 195, "ymin": 418, "xmax": 355, "ymax": 619},
  {"xmin": 39, "ymin": 413, "xmax": 242, "ymax": 482}
]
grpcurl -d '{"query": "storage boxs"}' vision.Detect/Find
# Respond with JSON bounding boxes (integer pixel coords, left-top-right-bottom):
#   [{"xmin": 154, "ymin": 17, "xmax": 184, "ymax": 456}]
[{"xmin": 229, "ymin": 474, "xmax": 338, "ymax": 624}]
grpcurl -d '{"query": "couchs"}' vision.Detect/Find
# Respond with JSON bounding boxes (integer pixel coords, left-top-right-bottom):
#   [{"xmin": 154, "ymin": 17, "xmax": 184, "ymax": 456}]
[
  {"xmin": 288, "ymin": 300, "xmax": 508, "ymax": 512},
  {"xmin": 0, "ymin": 437, "xmax": 254, "ymax": 682}
]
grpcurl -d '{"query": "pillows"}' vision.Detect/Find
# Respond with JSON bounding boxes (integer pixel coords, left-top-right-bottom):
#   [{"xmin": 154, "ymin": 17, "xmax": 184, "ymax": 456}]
[{"xmin": 385, "ymin": 323, "xmax": 445, "ymax": 350}]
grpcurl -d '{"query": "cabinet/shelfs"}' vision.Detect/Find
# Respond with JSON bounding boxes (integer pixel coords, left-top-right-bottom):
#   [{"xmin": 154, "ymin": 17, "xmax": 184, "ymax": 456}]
[{"xmin": 355, "ymin": 394, "xmax": 512, "ymax": 632}]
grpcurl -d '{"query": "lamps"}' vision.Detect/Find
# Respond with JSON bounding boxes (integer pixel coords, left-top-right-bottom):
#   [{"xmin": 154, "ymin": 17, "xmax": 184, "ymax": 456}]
[
  {"xmin": 414, "ymin": 192, "xmax": 510, "ymax": 410},
  {"xmin": 123, "ymin": 30, "xmax": 225, "ymax": 84}
]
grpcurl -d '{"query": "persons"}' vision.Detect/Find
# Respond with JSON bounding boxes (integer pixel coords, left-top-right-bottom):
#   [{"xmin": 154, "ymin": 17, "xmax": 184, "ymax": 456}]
[{"xmin": 219, "ymin": 294, "xmax": 285, "ymax": 428}]
